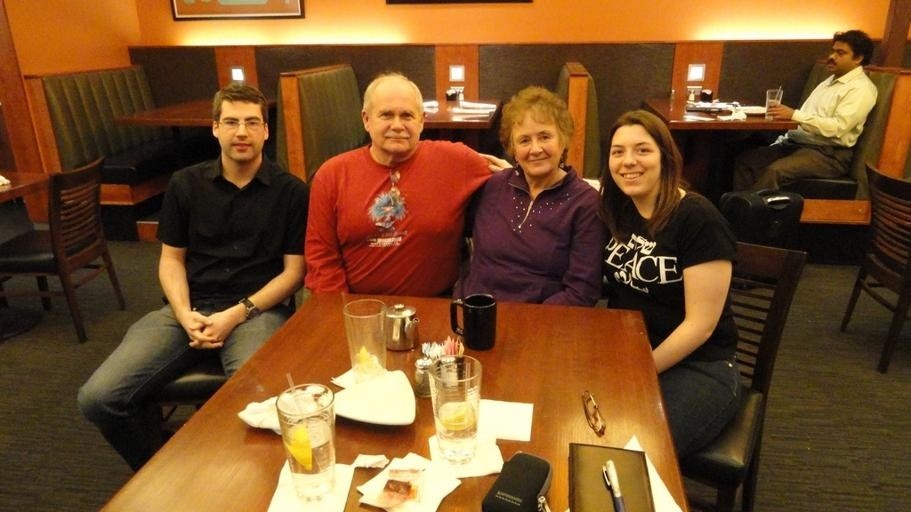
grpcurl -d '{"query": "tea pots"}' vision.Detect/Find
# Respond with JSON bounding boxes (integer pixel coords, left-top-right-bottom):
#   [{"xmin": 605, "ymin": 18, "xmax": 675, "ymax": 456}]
[{"xmin": 385, "ymin": 302, "xmax": 420, "ymax": 352}]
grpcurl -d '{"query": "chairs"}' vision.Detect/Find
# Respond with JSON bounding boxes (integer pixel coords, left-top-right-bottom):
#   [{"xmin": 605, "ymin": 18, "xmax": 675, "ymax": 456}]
[
  {"xmin": 1, "ymin": 148, "xmax": 126, "ymax": 347},
  {"xmin": 667, "ymin": 228, "xmax": 809, "ymax": 510},
  {"xmin": 837, "ymin": 159, "xmax": 911, "ymax": 375}
]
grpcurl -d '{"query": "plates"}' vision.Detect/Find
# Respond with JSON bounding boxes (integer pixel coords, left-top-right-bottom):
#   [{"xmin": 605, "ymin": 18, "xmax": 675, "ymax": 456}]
[
  {"xmin": 739, "ymin": 105, "xmax": 766, "ymax": 116},
  {"xmin": 334, "ymin": 365, "xmax": 418, "ymax": 427}
]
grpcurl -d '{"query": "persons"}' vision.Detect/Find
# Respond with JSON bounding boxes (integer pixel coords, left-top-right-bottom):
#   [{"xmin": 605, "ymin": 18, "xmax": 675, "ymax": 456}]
[
  {"xmin": 302, "ymin": 73, "xmax": 492, "ymax": 296},
  {"xmin": 77, "ymin": 83, "xmax": 309, "ymax": 474},
  {"xmin": 452, "ymin": 87, "xmax": 606, "ymax": 306},
  {"xmin": 479, "ymin": 109, "xmax": 745, "ymax": 467},
  {"xmin": 733, "ymin": 30, "xmax": 878, "ymax": 192}
]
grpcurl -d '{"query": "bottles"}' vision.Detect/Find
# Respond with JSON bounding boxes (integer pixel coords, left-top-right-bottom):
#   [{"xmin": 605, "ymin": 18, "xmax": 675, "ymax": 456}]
[
  {"xmin": 670, "ymin": 89, "xmax": 677, "ymax": 100},
  {"xmin": 688, "ymin": 90, "xmax": 697, "ymax": 101},
  {"xmin": 416, "ymin": 359, "xmax": 433, "ymax": 398},
  {"xmin": 458, "ymin": 89, "xmax": 465, "ymax": 102},
  {"xmin": 700, "ymin": 90, "xmax": 713, "ymax": 101},
  {"xmin": 447, "ymin": 89, "xmax": 456, "ymax": 101}
]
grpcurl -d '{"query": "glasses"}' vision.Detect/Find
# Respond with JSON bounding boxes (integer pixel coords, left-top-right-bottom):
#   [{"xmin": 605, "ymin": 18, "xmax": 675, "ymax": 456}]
[
  {"xmin": 217, "ymin": 119, "xmax": 264, "ymax": 131},
  {"xmin": 581, "ymin": 389, "xmax": 606, "ymax": 436},
  {"xmin": 390, "ymin": 168, "xmax": 401, "ymax": 206}
]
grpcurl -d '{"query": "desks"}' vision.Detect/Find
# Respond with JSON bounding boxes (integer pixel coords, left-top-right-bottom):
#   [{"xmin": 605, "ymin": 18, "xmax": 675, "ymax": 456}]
[{"xmin": 0, "ymin": 168, "xmax": 59, "ymax": 344}]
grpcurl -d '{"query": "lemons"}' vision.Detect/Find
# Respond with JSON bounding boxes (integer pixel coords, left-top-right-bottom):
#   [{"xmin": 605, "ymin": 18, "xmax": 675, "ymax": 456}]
[
  {"xmin": 283, "ymin": 424, "xmax": 314, "ymax": 472},
  {"xmin": 441, "ymin": 406, "xmax": 474, "ymax": 430},
  {"xmin": 359, "ymin": 346, "xmax": 369, "ymax": 364}
]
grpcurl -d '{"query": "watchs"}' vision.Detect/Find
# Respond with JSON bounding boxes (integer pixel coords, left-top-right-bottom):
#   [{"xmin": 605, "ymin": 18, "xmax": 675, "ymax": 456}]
[{"xmin": 238, "ymin": 297, "xmax": 259, "ymax": 320}]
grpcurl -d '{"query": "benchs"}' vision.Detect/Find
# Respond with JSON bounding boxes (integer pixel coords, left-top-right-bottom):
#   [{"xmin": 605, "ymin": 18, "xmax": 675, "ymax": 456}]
[{"xmin": 10, "ymin": 41, "xmax": 911, "ymax": 265}]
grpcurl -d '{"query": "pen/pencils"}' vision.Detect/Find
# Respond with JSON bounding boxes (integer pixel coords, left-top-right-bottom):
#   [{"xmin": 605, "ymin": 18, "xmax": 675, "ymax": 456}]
[{"xmin": 602, "ymin": 460, "xmax": 625, "ymax": 512}]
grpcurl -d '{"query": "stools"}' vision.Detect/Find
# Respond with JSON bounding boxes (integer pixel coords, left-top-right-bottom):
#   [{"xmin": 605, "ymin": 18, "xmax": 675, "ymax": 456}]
[{"xmin": 143, "ymin": 287, "xmax": 298, "ymax": 432}]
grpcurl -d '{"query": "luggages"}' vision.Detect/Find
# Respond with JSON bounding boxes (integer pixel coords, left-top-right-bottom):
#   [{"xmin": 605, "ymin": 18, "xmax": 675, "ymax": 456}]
[{"xmin": 720, "ymin": 187, "xmax": 804, "ymax": 291}]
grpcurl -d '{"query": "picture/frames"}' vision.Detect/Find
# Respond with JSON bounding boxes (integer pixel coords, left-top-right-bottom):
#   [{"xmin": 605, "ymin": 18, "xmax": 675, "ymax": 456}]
[{"xmin": 167, "ymin": 1, "xmax": 308, "ymax": 21}]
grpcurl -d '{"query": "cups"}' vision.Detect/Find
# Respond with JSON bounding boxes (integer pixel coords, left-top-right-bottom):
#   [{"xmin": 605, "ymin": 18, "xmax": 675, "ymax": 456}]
[
  {"xmin": 342, "ymin": 297, "xmax": 388, "ymax": 374},
  {"xmin": 765, "ymin": 90, "xmax": 785, "ymax": 121},
  {"xmin": 275, "ymin": 383, "xmax": 338, "ymax": 501},
  {"xmin": 430, "ymin": 354, "xmax": 482, "ymax": 463},
  {"xmin": 450, "ymin": 292, "xmax": 498, "ymax": 352}
]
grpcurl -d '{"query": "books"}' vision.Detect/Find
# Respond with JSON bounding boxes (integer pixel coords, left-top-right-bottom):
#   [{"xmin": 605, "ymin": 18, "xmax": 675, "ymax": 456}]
[{"xmin": 570, "ymin": 443, "xmax": 654, "ymax": 512}]
